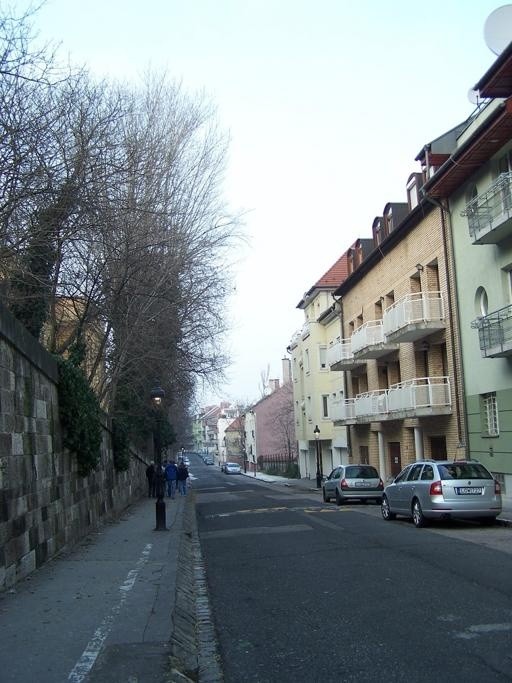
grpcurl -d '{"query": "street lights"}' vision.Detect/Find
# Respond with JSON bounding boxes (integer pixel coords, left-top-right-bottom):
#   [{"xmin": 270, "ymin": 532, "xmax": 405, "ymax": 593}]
[
  {"xmin": 147, "ymin": 378, "xmax": 172, "ymax": 532},
  {"xmin": 312, "ymin": 423, "xmax": 322, "ymax": 488}
]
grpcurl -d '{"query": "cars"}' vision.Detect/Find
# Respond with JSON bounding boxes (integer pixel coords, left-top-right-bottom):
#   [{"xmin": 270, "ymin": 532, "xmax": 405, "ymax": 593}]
[
  {"xmin": 322, "ymin": 463, "xmax": 385, "ymax": 506},
  {"xmin": 381, "ymin": 450, "xmax": 502, "ymax": 529},
  {"xmin": 177, "ymin": 448, "xmax": 214, "ymax": 466},
  {"xmin": 225, "ymin": 461, "xmax": 243, "ymax": 474},
  {"xmin": 221, "ymin": 462, "xmax": 226, "ymax": 471}
]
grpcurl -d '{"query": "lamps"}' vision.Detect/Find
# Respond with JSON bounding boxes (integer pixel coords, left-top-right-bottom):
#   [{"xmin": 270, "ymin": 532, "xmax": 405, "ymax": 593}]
[{"xmin": 414, "ymin": 262, "xmax": 425, "ymax": 272}]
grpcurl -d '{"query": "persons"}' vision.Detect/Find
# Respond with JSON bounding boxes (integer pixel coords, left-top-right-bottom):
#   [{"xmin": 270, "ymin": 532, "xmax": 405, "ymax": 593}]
[{"xmin": 146, "ymin": 459, "xmax": 189, "ymax": 499}]
